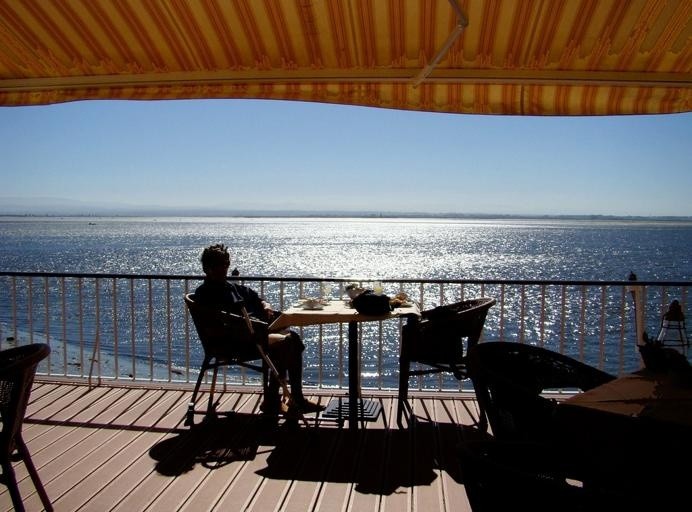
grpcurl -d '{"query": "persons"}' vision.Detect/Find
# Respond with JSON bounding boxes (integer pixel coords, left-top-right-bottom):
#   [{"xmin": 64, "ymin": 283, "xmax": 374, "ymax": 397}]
[{"xmin": 194, "ymin": 241, "xmax": 329, "ymax": 414}]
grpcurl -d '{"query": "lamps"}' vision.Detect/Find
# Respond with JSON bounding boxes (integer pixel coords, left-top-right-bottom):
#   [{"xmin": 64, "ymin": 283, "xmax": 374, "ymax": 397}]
[{"xmin": 649, "ymin": 298, "xmax": 689, "ymax": 360}]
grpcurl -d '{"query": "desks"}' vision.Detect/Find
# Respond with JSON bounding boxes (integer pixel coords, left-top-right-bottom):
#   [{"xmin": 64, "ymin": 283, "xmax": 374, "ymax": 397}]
[{"xmin": 554, "ymin": 375, "xmax": 690, "ymax": 511}]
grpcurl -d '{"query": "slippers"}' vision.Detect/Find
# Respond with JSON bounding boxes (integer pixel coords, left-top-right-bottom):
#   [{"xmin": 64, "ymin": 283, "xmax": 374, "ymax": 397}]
[
  {"xmin": 259, "ymin": 399, "xmax": 303, "ymax": 419},
  {"xmin": 286, "ymin": 400, "xmax": 327, "ymax": 412}
]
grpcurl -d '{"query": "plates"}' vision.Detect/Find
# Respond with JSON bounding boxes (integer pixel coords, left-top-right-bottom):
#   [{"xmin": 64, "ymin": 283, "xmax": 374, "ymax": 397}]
[
  {"xmin": 294, "ymin": 298, "xmax": 329, "ymax": 310},
  {"xmin": 345, "ymin": 282, "xmax": 408, "ymax": 308}
]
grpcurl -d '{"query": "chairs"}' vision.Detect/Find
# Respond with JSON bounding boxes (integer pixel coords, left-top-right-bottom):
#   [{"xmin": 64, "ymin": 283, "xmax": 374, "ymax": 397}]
[
  {"xmin": 466, "ymin": 337, "xmax": 612, "ymax": 512},
  {"xmin": 179, "ymin": 292, "xmax": 282, "ymax": 428},
  {"xmin": 0, "ymin": 343, "xmax": 54, "ymax": 512},
  {"xmin": 391, "ymin": 296, "xmax": 493, "ymax": 435}
]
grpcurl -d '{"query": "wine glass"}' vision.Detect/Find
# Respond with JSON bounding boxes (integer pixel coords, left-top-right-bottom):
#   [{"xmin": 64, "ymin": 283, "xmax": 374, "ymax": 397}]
[{"xmin": 322, "ymin": 282, "xmax": 331, "ymax": 305}]
